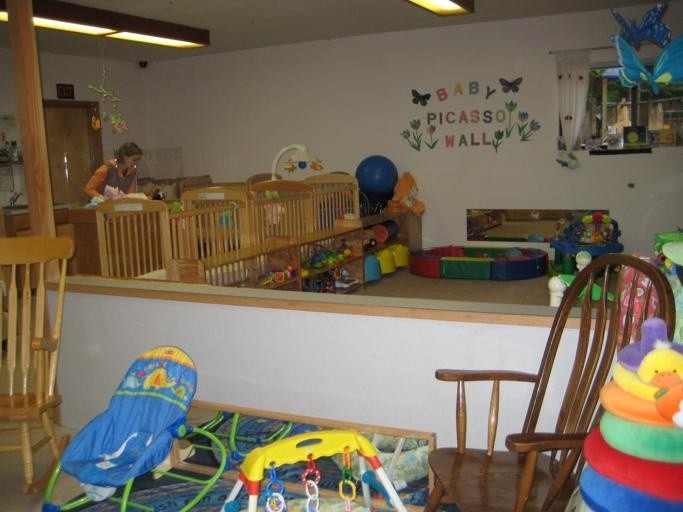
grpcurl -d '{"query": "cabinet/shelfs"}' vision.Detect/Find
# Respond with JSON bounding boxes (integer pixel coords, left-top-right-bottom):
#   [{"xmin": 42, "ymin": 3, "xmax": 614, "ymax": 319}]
[{"xmin": 200, "ymin": 210, "xmax": 422, "ymax": 293}]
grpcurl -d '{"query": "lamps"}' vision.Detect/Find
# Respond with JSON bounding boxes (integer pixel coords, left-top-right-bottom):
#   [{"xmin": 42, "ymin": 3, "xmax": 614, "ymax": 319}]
[
  {"xmin": 410, "ymin": 0, "xmax": 476, "ymax": 17},
  {"xmin": 271, "ymin": 143, "xmax": 320, "ymax": 181},
  {"xmin": 0, "ymin": 0, "xmax": 210, "ymax": 49}
]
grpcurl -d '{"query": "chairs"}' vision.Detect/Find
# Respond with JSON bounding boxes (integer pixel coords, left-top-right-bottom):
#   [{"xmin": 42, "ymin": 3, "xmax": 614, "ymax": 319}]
[
  {"xmin": 0, "ymin": 236, "xmax": 78, "ymax": 493},
  {"xmin": 422, "ymin": 253, "xmax": 675, "ymax": 511}
]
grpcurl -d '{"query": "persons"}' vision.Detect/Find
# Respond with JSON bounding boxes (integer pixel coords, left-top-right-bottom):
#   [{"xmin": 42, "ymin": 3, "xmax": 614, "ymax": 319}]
[
  {"xmin": 103, "ymin": 183, "xmax": 162, "ymax": 202},
  {"xmin": 83, "ymin": 141, "xmax": 145, "ymax": 207}
]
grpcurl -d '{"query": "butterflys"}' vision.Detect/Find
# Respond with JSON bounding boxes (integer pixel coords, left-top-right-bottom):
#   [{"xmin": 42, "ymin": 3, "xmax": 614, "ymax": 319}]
[
  {"xmin": 610, "ymin": 2, "xmax": 673, "ymax": 53},
  {"xmin": 411, "ymin": 89, "xmax": 433, "ymax": 105},
  {"xmin": 498, "ymin": 75, "xmax": 523, "ymax": 93},
  {"xmin": 608, "ymin": 29, "xmax": 682, "ymax": 96}
]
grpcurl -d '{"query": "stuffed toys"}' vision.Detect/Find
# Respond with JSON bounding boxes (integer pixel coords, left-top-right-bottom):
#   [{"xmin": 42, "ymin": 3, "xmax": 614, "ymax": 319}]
[
  {"xmin": 384, "ymin": 172, "xmax": 426, "ymax": 216},
  {"xmin": 570, "ymin": 315, "xmax": 683, "ymax": 511}
]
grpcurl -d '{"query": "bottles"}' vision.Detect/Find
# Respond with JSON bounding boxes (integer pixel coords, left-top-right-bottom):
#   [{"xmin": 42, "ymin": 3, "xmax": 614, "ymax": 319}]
[{"xmin": 5, "ymin": 140, "xmax": 19, "ymax": 163}]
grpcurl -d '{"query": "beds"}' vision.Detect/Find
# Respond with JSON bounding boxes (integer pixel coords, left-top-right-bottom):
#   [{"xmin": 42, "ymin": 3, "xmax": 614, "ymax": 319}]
[{"xmin": 68, "ymin": 171, "xmax": 361, "ymax": 288}]
